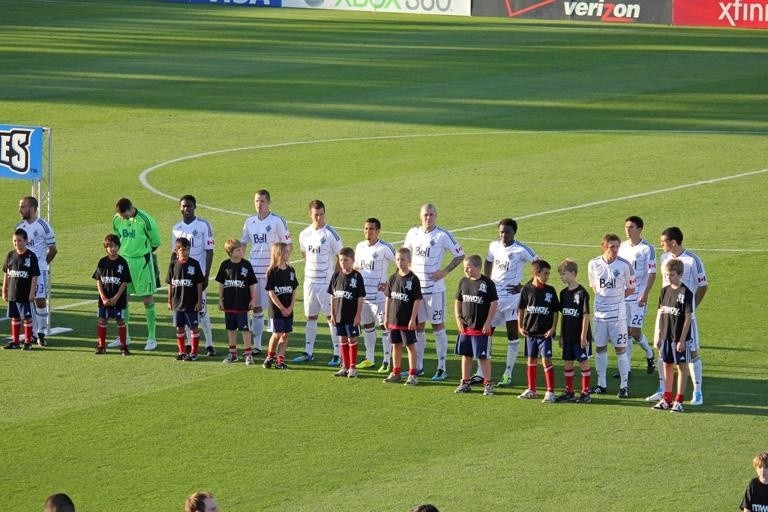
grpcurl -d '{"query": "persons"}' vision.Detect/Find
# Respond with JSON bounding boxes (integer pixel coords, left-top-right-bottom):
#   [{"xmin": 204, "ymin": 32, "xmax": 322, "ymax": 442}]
[
  {"xmin": 167, "ymin": 188, "xmax": 709, "ymax": 413},
  {"xmin": 108, "ymin": 198, "xmax": 162, "ymax": 350},
  {"xmin": 15, "ymin": 198, "xmax": 58, "ymax": 346},
  {"xmin": 42, "ymin": 492, "xmax": 78, "ymax": 511},
  {"xmin": 414, "ymin": 504, "xmax": 439, "ymax": 511},
  {"xmin": 739, "ymin": 450, "xmax": 768, "ymax": 511},
  {"xmin": 3, "ymin": 227, "xmax": 42, "ymax": 350},
  {"xmin": 185, "ymin": 491, "xmax": 222, "ymax": 512},
  {"xmin": 90, "ymin": 232, "xmax": 133, "ymax": 356}
]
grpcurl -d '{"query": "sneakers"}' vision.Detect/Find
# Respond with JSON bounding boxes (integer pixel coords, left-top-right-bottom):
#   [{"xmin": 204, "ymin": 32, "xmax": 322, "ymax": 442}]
[
  {"xmin": 455, "ymin": 375, "xmax": 493, "ymax": 395},
  {"xmin": 647, "ymin": 349, "xmax": 656, "ymax": 374},
  {"xmin": 328, "ymin": 355, "xmax": 374, "ymax": 378},
  {"xmin": 145, "ymin": 339, "xmax": 156, "ymax": 350},
  {"xmin": 517, "ymin": 388, "xmax": 536, "ymax": 398},
  {"xmin": 263, "ymin": 356, "xmax": 289, "ymax": 369},
  {"xmin": 377, "ymin": 362, "xmax": 423, "ymax": 386},
  {"xmin": 294, "ymin": 353, "xmax": 314, "ymax": 362},
  {"xmin": 96, "ymin": 336, "xmax": 131, "ymax": 356},
  {"xmin": 542, "ymin": 385, "xmax": 607, "ymax": 403},
  {"xmin": 176, "ymin": 345, "xmax": 217, "ymax": 361},
  {"xmin": 692, "ymin": 391, "xmax": 703, "ymax": 405},
  {"xmin": 3, "ymin": 333, "xmax": 47, "ymax": 350},
  {"xmin": 432, "ymin": 369, "xmax": 448, "ymax": 381},
  {"xmin": 618, "ymin": 387, "xmax": 631, "ymax": 398},
  {"xmin": 497, "ymin": 375, "xmax": 511, "ymax": 386},
  {"xmin": 223, "ymin": 348, "xmax": 259, "ymax": 365},
  {"xmin": 646, "ymin": 392, "xmax": 684, "ymax": 412}
]
